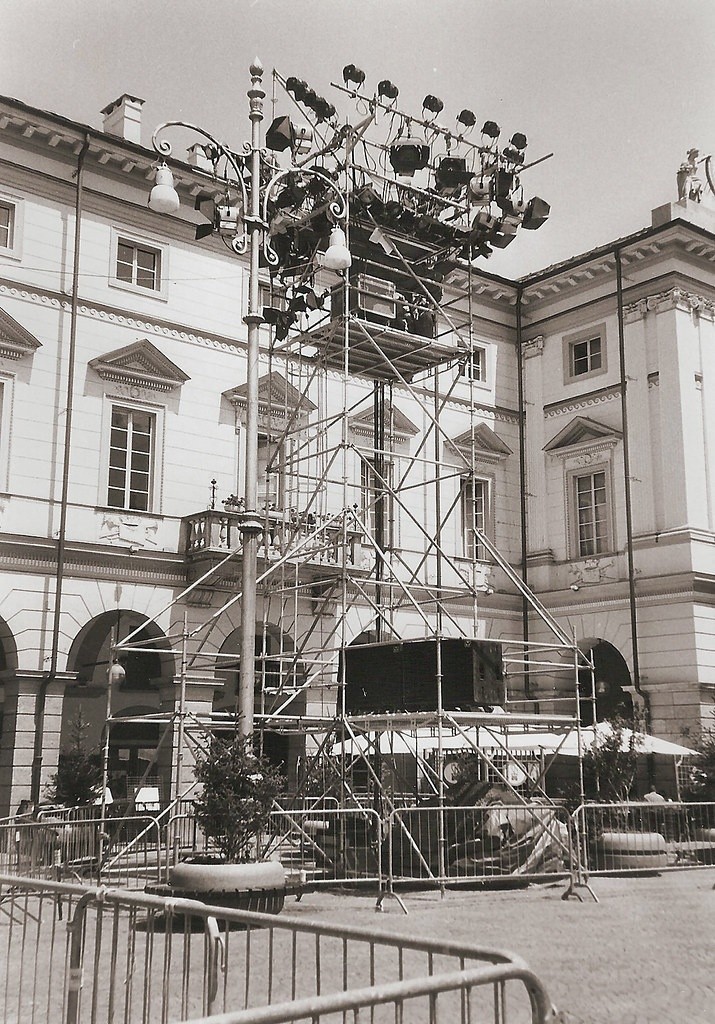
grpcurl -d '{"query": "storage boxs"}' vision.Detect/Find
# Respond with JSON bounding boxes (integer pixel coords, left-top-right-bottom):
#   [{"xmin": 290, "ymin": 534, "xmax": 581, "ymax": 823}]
[{"xmin": 331, "ymin": 273, "xmax": 397, "ymax": 323}]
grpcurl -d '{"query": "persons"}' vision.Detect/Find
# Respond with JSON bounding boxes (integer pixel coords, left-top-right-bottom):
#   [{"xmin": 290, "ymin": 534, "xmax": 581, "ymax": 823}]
[
  {"xmin": 643, "ymin": 784, "xmax": 666, "ymax": 831},
  {"xmin": 89, "ymin": 771, "xmax": 115, "ymax": 806},
  {"xmin": 676, "ymin": 146, "xmax": 712, "ymax": 202}
]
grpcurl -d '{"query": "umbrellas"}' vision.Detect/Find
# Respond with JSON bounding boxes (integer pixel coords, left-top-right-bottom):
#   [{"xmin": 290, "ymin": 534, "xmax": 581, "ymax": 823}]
[{"xmin": 313, "ymin": 711, "xmax": 705, "ymax": 794}]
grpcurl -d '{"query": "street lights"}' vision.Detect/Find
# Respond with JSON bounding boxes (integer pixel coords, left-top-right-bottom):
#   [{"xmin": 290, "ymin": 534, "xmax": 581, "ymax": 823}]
[
  {"xmin": 148, "ymin": 56, "xmax": 354, "ymax": 854},
  {"xmin": 98, "ymin": 627, "xmax": 126, "ymax": 864}
]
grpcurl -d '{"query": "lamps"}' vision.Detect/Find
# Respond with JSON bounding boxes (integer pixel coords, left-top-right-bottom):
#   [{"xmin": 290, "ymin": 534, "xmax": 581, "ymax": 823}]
[
  {"xmin": 195, "ymin": 65, "xmax": 550, "ymax": 336},
  {"xmin": 106, "ymin": 664, "xmax": 126, "ymax": 684},
  {"xmin": 148, "ymin": 156, "xmax": 180, "ymax": 213}
]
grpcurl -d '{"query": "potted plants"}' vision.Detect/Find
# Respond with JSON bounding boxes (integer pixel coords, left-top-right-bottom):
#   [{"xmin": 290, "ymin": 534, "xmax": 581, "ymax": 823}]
[
  {"xmin": 168, "ymin": 711, "xmax": 284, "ymax": 930},
  {"xmin": 691, "ymin": 714, "xmax": 714, "ymax": 863},
  {"xmin": 591, "ymin": 703, "xmax": 670, "ymax": 874}
]
